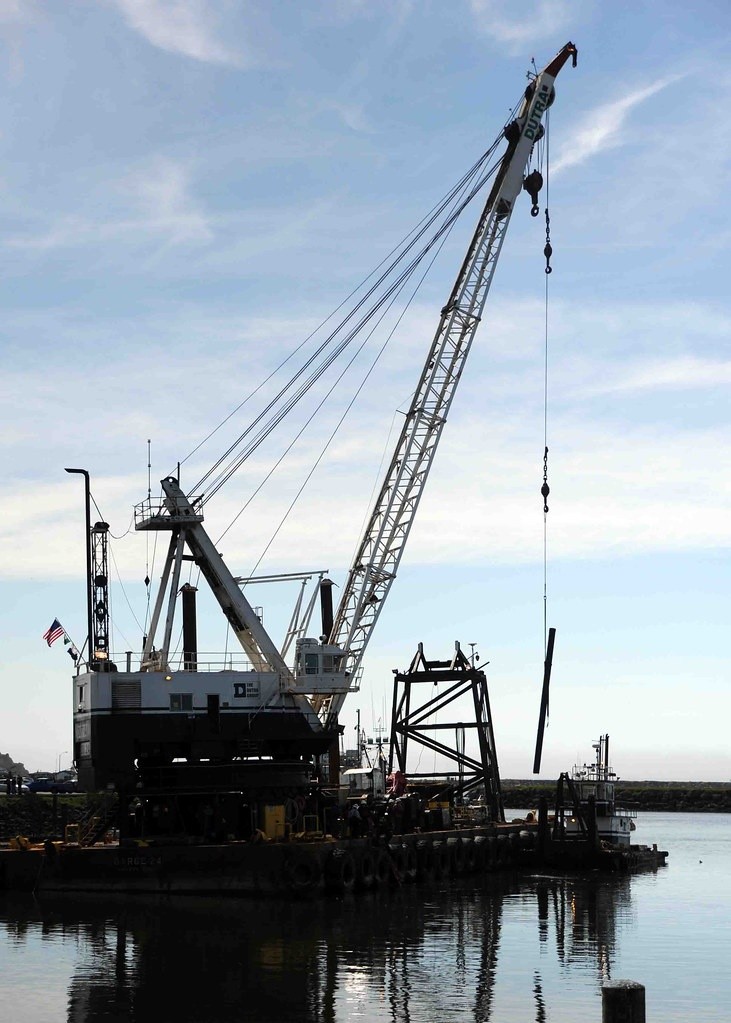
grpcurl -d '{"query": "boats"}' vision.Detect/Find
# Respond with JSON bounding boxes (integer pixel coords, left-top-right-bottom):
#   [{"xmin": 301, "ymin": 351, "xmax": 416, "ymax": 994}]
[{"xmin": 529, "ymin": 734, "xmax": 670, "ymax": 866}]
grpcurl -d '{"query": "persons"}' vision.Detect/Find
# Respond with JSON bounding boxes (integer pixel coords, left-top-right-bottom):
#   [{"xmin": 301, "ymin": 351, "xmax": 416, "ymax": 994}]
[
  {"xmin": 4, "ymin": 777, "xmax": 23, "ymax": 795},
  {"xmin": 341, "ymin": 797, "xmax": 404, "ymax": 834}
]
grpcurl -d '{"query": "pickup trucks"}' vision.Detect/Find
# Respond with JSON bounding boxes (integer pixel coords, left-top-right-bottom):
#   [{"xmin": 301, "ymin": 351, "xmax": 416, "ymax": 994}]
[{"xmin": 26, "ymin": 778, "xmax": 70, "ymax": 794}]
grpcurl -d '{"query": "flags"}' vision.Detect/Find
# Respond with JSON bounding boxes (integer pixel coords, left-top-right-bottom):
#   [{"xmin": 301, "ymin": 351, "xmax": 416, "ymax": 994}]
[
  {"xmin": 43, "ymin": 620, "xmax": 64, "ymax": 648},
  {"xmin": 67, "ymin": 647, "xmax": 77, "ymax": 660},
  {"xmin": 64, "ymin": 634, "xmax": 70, "ymax": 645}
]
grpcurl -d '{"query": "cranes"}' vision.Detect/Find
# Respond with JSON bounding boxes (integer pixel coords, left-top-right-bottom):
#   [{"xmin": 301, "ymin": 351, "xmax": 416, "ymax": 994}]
[{"xmin": 74, "ymin": 41, "xmax": 580, "ymax": 797}]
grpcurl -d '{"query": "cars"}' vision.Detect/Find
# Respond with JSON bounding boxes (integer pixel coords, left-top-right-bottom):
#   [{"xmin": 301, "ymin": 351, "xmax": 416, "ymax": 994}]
[{"xmin": 0, "ymin": 779, "xmax": 29, "ymax": 794}]
